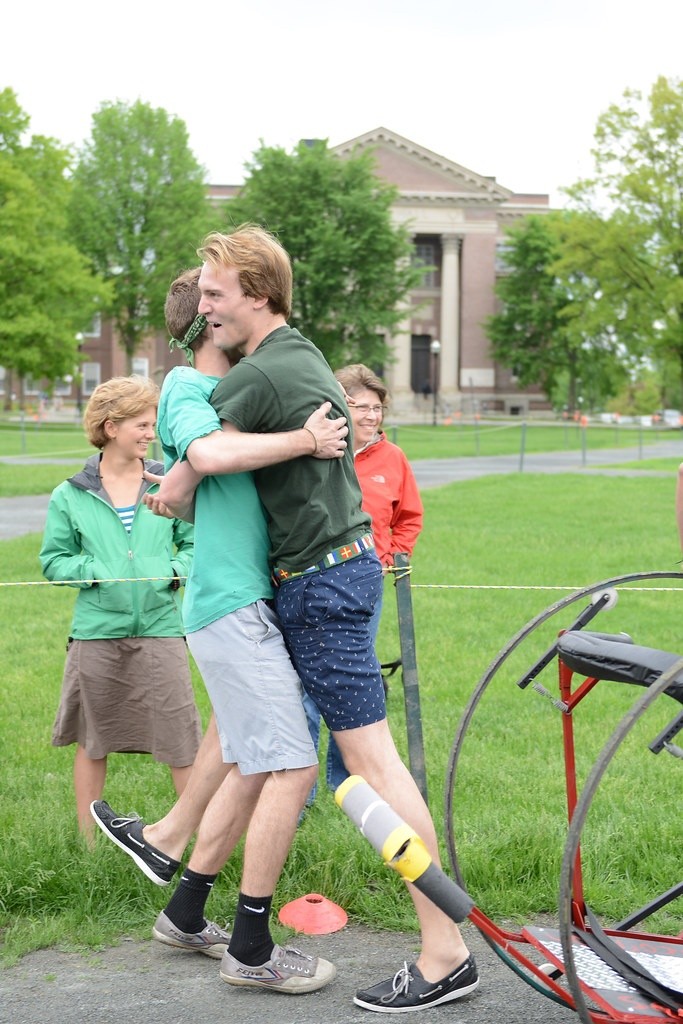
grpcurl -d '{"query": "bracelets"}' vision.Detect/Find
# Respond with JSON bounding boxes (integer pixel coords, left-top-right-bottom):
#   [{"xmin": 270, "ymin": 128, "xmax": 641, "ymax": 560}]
[{"xmin": 304, "ymin": 427, "xmax": 318, "ymax": 455}]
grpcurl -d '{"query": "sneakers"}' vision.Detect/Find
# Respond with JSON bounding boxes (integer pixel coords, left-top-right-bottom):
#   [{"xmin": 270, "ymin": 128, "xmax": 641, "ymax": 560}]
[
  {"xmin": 353, "ymin": 952, "xmax": 480, "ymax": 1013},
  {"xmin": 90, "ymin": 800, "xmax": 181, "ymax": 886},
  {"xmin": 153, "ymin": 909, "xmax": 233, "ymax": 959},
  {"xmin": 219, "ymin": 943, "xmax": 337, "ymax": 993}
]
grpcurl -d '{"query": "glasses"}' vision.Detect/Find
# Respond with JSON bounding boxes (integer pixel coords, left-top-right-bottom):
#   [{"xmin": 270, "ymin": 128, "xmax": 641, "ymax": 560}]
[{"xmin": 348, "ymin": 403, "xmax": 389, "ymax": 416}]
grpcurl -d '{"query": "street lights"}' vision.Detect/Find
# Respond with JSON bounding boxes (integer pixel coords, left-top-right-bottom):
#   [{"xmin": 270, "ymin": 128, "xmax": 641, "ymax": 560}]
[
  {"xmin": 75, "ymin": 332, "xmax": 84, "ymax": 416},
  {"xmin": 430, "ymin": 339, "xmax": 441, "ymax": 427}
]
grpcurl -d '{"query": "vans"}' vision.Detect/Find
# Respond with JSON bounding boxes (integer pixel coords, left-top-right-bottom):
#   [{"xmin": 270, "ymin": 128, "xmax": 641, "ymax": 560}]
[{"xmin": 653, "ymin": 409, "xmax": 682, "ymax": 427}]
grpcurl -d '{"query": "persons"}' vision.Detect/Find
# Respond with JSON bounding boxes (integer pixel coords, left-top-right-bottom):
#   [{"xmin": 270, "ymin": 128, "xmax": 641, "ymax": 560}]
[
  {"xmin": 274, "ymin": 359, "xmax": 424, "ymax": 829},
  {"xmin": 39, "ymin": 375, "xmax": 202, "ymax": 877},
  {"xmin": 154, "ymin": 262, "xmax": 348, "ymax": 994},
  {"xmin": 91, "ymin": 225, "xmax": 481, "ymax": 1012}
]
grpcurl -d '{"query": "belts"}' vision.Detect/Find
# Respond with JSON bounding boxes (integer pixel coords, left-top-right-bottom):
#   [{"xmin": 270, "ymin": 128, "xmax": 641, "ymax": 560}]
[{"xmin": 273, "ymin": 533, "xmax": 375, "ymax": 582}]
